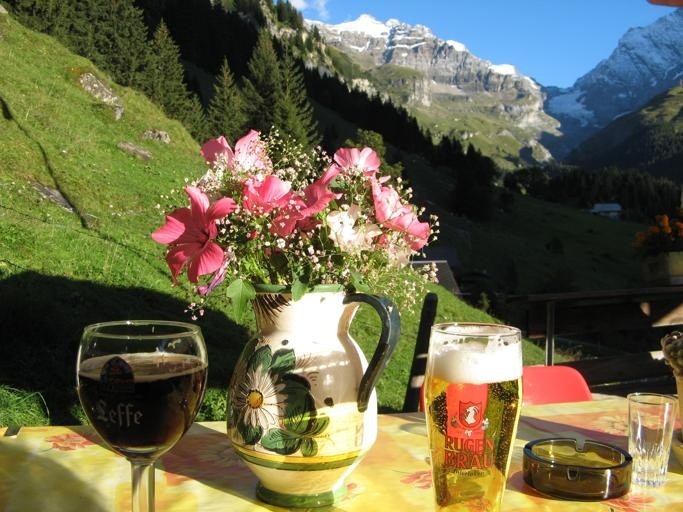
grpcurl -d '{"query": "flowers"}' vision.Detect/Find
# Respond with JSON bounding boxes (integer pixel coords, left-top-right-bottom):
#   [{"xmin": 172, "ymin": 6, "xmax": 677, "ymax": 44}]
[{"xmin": 146, "ymin": 119, "xmax": 444, "ymax": 322}]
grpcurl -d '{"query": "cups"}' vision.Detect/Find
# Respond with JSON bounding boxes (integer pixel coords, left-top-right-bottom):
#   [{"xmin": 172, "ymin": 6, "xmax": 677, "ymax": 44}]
[
  {"xmin": 627, "ymin": 393, "xmax": 679, "ymax": 495},
  {"xmin": 423, "ymin": 322, "xmax": 522, "ymax": 512}
]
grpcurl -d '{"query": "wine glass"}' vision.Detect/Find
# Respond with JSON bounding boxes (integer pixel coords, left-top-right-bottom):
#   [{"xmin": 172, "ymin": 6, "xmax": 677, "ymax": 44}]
[{"xmin": 77, "ymin": 319, "xmax": 209, "ymax": 512}]
